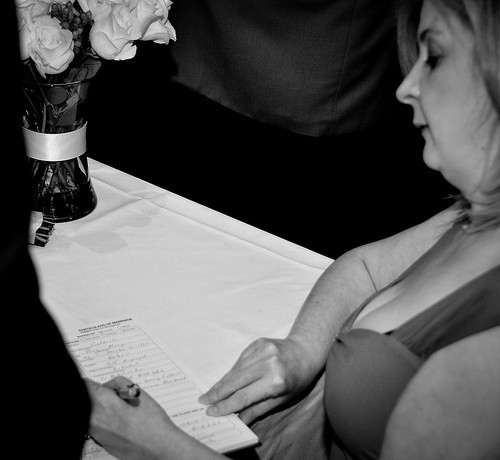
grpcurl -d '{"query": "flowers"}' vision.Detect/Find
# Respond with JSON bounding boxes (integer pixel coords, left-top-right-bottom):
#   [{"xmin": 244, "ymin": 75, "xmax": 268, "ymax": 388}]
[{"xmin": 16, "ymin": 0, "xmax": 178, "ymax": 83}]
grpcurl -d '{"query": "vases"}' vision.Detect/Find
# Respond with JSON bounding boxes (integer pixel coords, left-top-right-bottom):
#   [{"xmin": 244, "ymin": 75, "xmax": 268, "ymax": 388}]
[{"xmin": 19, "ymin": 81, "xmax": 99, "ymax": 224}]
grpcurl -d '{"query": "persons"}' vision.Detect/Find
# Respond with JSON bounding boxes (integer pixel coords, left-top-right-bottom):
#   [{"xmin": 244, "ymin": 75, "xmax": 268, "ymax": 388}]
[
  {"xmin": 142, "ymin": 4, "xmax": 459, "ymax": 260},
  {"xmin": 83, "ymin": 3, "xmax": 500, "ymax": 459}
]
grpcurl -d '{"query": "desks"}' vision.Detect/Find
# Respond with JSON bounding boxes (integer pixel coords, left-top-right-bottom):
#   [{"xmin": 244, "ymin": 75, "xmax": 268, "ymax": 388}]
[{"xmin": 26, "ymin": 155, "xmax": 339, "ymax": 460}]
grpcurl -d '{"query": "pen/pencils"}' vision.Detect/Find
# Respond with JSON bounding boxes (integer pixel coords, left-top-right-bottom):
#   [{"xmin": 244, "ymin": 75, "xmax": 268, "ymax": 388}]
[{"xmin": 113, "ymin": 383, "xmax": 141, "ymax": 399}]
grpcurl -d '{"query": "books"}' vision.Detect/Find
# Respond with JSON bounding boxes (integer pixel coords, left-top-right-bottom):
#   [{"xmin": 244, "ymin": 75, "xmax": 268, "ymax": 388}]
[{"xmin": 60, "ymin": 314, "xmax": 261, "ymax": 460}]
